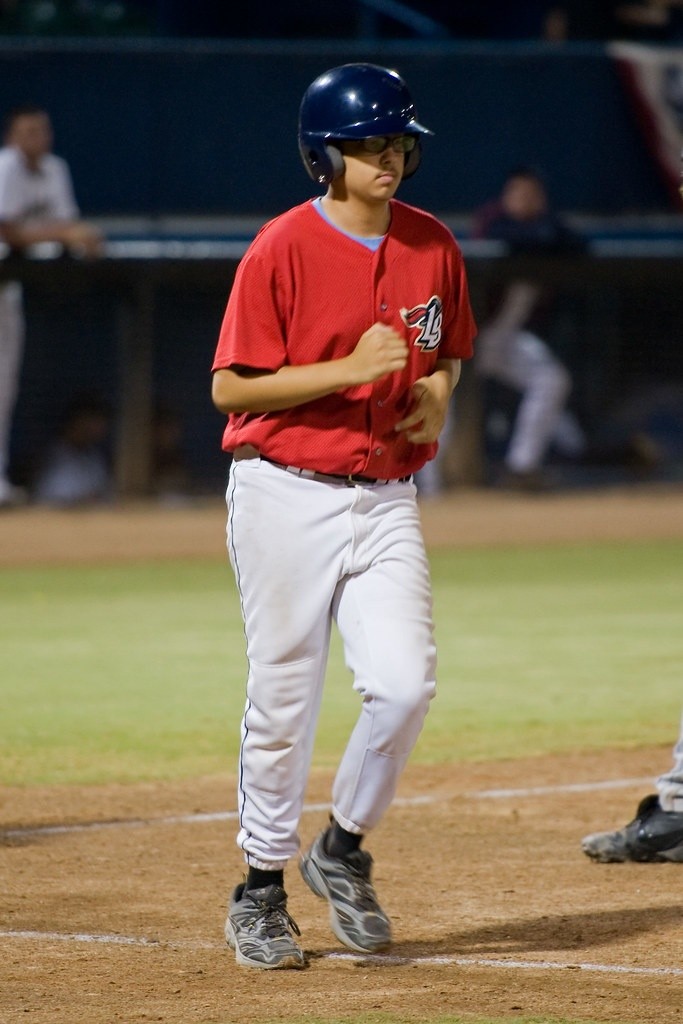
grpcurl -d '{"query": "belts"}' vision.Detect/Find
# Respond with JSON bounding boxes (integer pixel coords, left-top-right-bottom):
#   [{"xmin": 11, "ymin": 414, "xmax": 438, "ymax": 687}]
[{"xmin": 259, "ymin": 453, "xmax": 411, "ymax": 483}]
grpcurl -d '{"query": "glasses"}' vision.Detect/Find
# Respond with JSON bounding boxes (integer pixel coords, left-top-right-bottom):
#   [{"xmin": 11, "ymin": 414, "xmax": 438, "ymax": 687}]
[{"xmin": 342, "ymin": 135, "xmax": 418, "ymax": 155}]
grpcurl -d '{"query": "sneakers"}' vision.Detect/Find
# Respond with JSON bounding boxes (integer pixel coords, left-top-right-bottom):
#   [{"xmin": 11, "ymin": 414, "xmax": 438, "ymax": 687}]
[
  {"xmin": 223, "ymin": 882, "xmax": 306, "ymax": 969},
  {"xmin": 580, "ymin": 793, "xmax": 683, "ymax": 865},
  {"xmin": 299, "ymin": 830, "xmax": 394, "ymax": 952}
]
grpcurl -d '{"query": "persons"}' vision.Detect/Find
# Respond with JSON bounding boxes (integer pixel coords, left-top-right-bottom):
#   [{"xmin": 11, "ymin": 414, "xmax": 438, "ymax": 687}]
[
  {"xmin": 213, "ymin": 64, "xmax": 478, "ymax": 970},
  {"xmin": 1, "ymin": 108, "xmax": 103, "ymax": 514},
  {"xmin": 474, "ymin": 173, "xmax": 604, "ymax": 472}
]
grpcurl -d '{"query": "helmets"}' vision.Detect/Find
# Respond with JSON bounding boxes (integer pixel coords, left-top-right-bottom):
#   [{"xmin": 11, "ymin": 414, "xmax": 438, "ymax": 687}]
[{"xmin": 299, "ymin": 63, "xmax": 435, "ymax": 185}]
